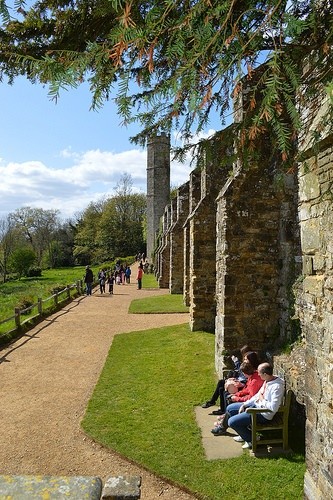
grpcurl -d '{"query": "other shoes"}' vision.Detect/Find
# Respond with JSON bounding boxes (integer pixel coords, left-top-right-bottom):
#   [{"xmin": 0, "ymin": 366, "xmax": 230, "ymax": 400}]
[
  {"xmin": 202, "ymin": 401, "xmax": 216, "ymax": 408},
  {"xmin": 211, "ymin": 425, "xmax": 226, "ymax": 436},
  {"xmin": 233, "ymin": 435, "xmax": 245, "ymax": 441},
  {"xmin": 214, "ymin": 422, "xmax": 225, "ymax": 427},
  {"xmin": 217, "ymin": 415, "xmax": 225, "ymax": 422},
  {"xmin": 241, "ymin": 441, "xmax": 251, "ymax": 449},
  {"xmin": 213, "ymin": 409, "xmax": 225, "ymax": 415}
]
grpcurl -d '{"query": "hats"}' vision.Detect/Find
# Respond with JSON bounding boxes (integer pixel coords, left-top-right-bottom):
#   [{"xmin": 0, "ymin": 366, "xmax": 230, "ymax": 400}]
[{"xmin": 86, "ymin": 266, "xmax": 89, "ymax": 269}]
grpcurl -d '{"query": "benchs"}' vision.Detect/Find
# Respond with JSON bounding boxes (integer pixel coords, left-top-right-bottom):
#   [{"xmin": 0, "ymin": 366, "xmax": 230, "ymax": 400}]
[{"xmin": 221, "ymin": 370, "xmax": 292, "ymax": 453}]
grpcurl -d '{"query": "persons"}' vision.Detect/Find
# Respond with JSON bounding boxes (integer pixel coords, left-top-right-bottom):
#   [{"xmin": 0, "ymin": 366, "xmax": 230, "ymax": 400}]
[
  {"xmin": 202, "ymin": 345, "xmax": 264, "ymax": 435},
  {"xmin": 226, "ymin": 363, "xmax": 284, "ymax": 449},
  {"xmin": 135, "ymin": 252, "xmax": 149, "ymax": 290},
  {"xmin": 84, "ymin": 266, "xmax": 93, "ymax": 295},
  {"xmin": 98, "ymin": 259, "xmax": 131, "ymax": 294}
]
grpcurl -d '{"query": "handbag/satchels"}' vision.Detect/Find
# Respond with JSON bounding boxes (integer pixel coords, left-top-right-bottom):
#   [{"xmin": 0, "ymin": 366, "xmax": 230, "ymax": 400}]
[
  {"xmin": 101, "ymin": 280, "xmax": 105, "ymax": 285},
  {"xmin": 116, "ymin": 277, "xmax": 120, "ymax": 282}
]
